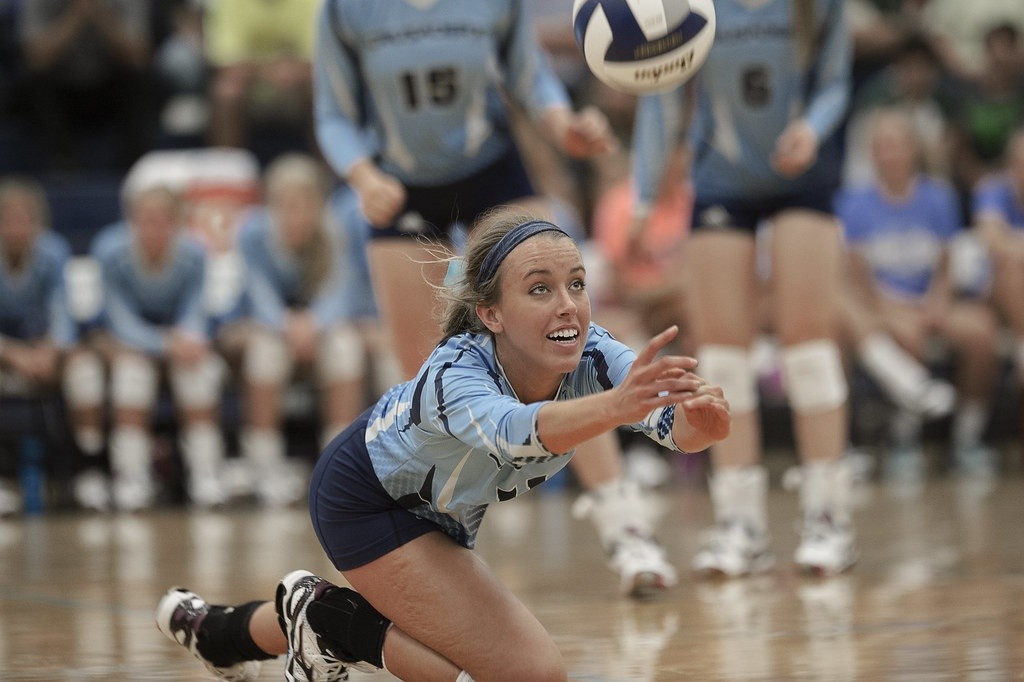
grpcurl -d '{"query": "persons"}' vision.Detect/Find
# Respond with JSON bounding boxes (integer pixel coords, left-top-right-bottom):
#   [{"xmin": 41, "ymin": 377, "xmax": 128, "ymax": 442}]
[
  {"xmin": 624, "ymin": 0, "xmax": 862, "ymax": 582},
  {"xmin": 0, "ymin": 0, "xmax": 1024, "ymax": 518},
  {"xmin": 154, "ymin": 204, "xmax": 732, "ymax": 682},
  {"xmin": 314, "ymin": 0, "xmax": 681, "ymax": 598}
]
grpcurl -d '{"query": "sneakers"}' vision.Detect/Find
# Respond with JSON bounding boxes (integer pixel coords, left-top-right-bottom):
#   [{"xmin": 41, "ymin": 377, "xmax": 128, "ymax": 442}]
[
  {"xmin": 794, "ymin": 513, "xmax": 858, "ymax": 575},
  {"xmin": 275, "ymin": 569, "xmax": 382, "ymax": 682},
  {"xmin": 156, "ymin": 586, "xmax": 260, "ymax": 682},
  {"xmin": 690, "ymin": 519, "xmax": 777, "ymax": 577},
  {"xmin": 603, "ymin": 530, "xmax": 678, "ymax": 596}
]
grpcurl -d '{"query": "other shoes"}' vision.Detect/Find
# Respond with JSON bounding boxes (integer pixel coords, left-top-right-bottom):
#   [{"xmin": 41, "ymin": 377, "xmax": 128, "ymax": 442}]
[
  {"xmin": 889, "ymin": 454, "xmax": 928, "ymax": 500},
  {"xmin": 0, "ymin": 431, "xmax": 305, "ymax": 509},
  {"xmin": 918, "ymin": 380, "xmax": 957, "ymax": 418},
  {"xmin": 946, "ymin": 447, "xmax": 1006, "ymax": 498}
]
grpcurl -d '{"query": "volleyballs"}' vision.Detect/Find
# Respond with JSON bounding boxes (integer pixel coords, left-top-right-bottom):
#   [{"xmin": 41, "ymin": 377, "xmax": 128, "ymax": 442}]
[{"xmin": 571, "ymin": 0, "xmax": 716, "ymax": 96}]
